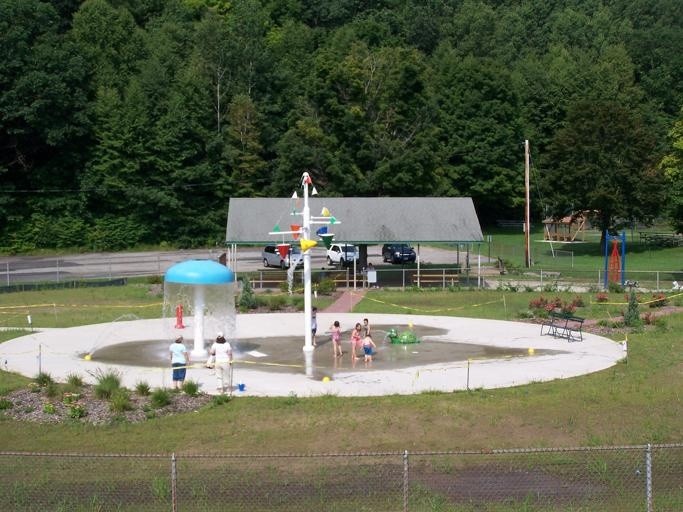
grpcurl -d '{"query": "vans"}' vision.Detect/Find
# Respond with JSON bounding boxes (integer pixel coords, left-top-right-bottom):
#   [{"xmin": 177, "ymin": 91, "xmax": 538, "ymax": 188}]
[{"xmin": 262, "ymin": 244, "xmax": 303, "ymax": 269}]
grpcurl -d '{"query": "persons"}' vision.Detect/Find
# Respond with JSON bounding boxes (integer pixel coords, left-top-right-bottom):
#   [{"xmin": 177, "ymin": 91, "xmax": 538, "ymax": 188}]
[
  {"xmin": 311, "ymin": 306, "xmax": 317, "ymax": 346},
  {"xmin": 366, "ymin": 263, "xmax": 379, "ymax": 289},
  {"xmin": 208, "ymin": 330, "xmax": 234, "ymax": 396},
  {"xmin": 169, "ymin": 335, "xmax": 190, "ymax": 392},
  {"xmin": 329, "ymin": 318, "xmax": 377, "ymax": 362}
]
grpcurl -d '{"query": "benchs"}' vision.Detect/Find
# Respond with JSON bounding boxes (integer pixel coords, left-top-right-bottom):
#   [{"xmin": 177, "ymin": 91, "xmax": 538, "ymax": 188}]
[
  {"xmin": 544, "ymin": 313, "xmax": 582, "ymax": 342},
  {"xmin": 411, "ymin": 273, "xmax": 460, "ymax": 287},
  {"xmin": 323, "ymin": 274, "xmax": 374, "ymax": 288},
  {"xmin": 247, "ymin": 272, "xmax": 289, "ymax": 289}
]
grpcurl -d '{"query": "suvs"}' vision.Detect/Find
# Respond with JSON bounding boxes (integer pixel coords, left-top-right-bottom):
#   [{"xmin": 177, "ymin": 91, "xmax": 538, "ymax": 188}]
[
  {"xmin": 381, "ymin": 244, "xmax": 416, "ymax": 264},
  {"xmin": 325, "ymin": 243, "xmax": 360, "ymax": 267}
]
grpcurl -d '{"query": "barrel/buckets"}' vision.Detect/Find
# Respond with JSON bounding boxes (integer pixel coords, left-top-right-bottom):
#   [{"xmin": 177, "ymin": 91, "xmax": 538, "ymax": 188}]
[{"xmin": 238, "ymin": 380, "xmax": 245, "ymax": 391}]
